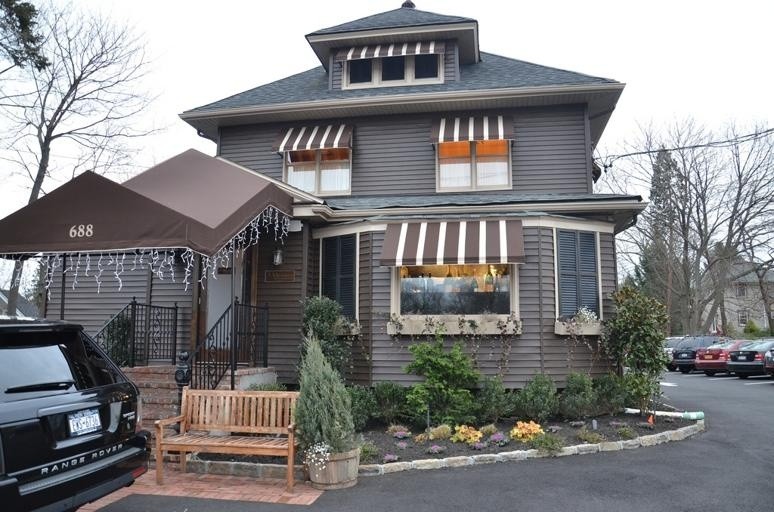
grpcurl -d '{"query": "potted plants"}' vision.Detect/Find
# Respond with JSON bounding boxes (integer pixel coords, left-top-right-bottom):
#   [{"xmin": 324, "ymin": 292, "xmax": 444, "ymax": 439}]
[{"xmin": 292, "ymin": 327, "xmax": 361, "ymax": 490}]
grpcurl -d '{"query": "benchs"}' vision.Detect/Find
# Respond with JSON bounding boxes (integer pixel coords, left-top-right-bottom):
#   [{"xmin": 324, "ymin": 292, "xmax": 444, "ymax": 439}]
[{"xmin": 153, "ymin": 384, "xmax": 301, "ymax": 493}]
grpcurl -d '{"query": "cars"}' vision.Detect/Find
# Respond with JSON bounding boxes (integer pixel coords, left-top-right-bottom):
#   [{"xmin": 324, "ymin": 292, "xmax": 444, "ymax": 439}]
[
  {"xmin": 0, "ymin": 318, "xmax": 152, "ymax": 512},
  {"xmin": 663, "ymin": 336, "xmax": 774, "ymax": 379}
]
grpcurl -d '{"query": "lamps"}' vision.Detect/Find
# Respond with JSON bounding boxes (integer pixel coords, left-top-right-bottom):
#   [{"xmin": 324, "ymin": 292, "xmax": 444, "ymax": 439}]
[{"xmin": 271, "ymin": 246, "xmax": 284, "ymax": 266}]
